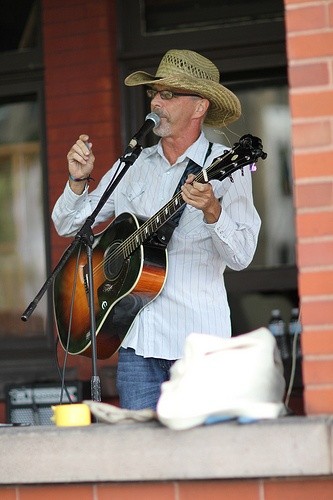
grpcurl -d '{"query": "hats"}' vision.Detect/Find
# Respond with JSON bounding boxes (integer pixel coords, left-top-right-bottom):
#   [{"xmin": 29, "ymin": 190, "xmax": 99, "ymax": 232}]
[{"xmin": 125, "ymin": 49, "xmax": 241, "ymax": 127}]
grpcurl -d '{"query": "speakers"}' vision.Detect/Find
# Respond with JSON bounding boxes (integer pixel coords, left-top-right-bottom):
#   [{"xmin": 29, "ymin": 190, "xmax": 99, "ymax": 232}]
[{"xmin": 6, "ymin": 379, "xmax": 96, "ymax": 427}]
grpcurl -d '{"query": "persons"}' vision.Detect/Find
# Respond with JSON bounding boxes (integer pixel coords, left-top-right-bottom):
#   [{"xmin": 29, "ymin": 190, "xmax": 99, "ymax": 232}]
[{"xmin": 50, "ymin": 50, "xmax": 261, "ymax": 411}]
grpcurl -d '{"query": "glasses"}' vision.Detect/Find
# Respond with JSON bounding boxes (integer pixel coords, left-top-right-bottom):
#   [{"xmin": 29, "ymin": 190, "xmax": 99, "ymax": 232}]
[{"xmin": 146, "ymin": 87, "xmax": 205, "ymax": 100}]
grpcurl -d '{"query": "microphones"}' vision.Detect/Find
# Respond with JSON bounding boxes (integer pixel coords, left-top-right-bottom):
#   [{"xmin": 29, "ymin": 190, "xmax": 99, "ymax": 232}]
[{"xmin": 126, "ymin": 113, "xmax": 159, "ymax": 153}]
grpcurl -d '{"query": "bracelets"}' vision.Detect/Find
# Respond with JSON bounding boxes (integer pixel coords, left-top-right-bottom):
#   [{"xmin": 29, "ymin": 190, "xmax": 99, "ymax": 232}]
[{"xmin": 69, "ymin": 174, "xmax": 96, "ymax": 183}]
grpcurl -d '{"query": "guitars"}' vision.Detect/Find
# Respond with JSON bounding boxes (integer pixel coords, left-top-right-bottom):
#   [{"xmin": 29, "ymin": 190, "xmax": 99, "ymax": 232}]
[{"xmin": 52, "ymin": 134, "xmax": 267, "ymax": 362}]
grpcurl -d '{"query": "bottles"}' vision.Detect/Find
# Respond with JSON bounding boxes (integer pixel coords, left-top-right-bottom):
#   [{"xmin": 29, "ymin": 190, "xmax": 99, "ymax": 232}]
[
  {"xmin": 267, "ymin": 310, "xmax": 288, "ymax": 361},
  {"xmin": 287, "ymin": 307, "xmax": 302, "ymax": 359}
]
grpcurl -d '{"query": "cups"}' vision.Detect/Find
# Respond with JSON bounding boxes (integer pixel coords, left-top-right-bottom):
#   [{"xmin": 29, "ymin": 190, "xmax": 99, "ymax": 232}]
[{"xmin": 54, "ymin": 404, "xmax": 91, "ymax": 426}]
